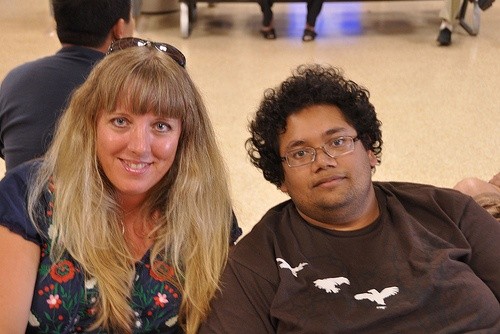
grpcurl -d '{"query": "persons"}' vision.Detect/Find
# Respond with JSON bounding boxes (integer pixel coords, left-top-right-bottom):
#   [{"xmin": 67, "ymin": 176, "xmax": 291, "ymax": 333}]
[
  {"xmin": 437, "ymin": 0, "xmax": 495, "ymax": 45},
  {"xmin": 196, "ymin": 64, "xmax": 500, "ymax": 334},
  {"xmin": 0, "ymin": 0, "xmax": 242, "ymax": 255},
  {"xmin": 256, "ymin": 0, "xmax": 324, "ymax": 41},
  {"xmin": 0, "ymin": 38, "xmax": 233, "ymax": 334}
]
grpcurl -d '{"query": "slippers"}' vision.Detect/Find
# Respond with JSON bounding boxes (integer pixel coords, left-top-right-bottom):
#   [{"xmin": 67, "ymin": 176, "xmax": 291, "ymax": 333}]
[
  {"xmin": 261, "ymin": 28, "xmax": 277, "ymax": 39},
  {"xmin": 303, "ymin": 29, "xmax": 316, "ymax": 41}
]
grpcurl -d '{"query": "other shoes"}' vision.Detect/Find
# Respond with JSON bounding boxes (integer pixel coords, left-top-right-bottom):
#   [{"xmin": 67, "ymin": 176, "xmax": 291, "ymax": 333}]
[{"xmin": 438, "ymin": 28, "xmax": 452, "ymax": 46}]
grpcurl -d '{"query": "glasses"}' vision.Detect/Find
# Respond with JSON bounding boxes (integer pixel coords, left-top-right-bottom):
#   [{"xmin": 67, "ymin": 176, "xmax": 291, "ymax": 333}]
[
  {"xmin": 106, "ymin": 37, "xmax": 186, "ymax": 69},
  {"xmin": 277, "ymin": 132, "xmax": 362, "ymax": 168}
]
